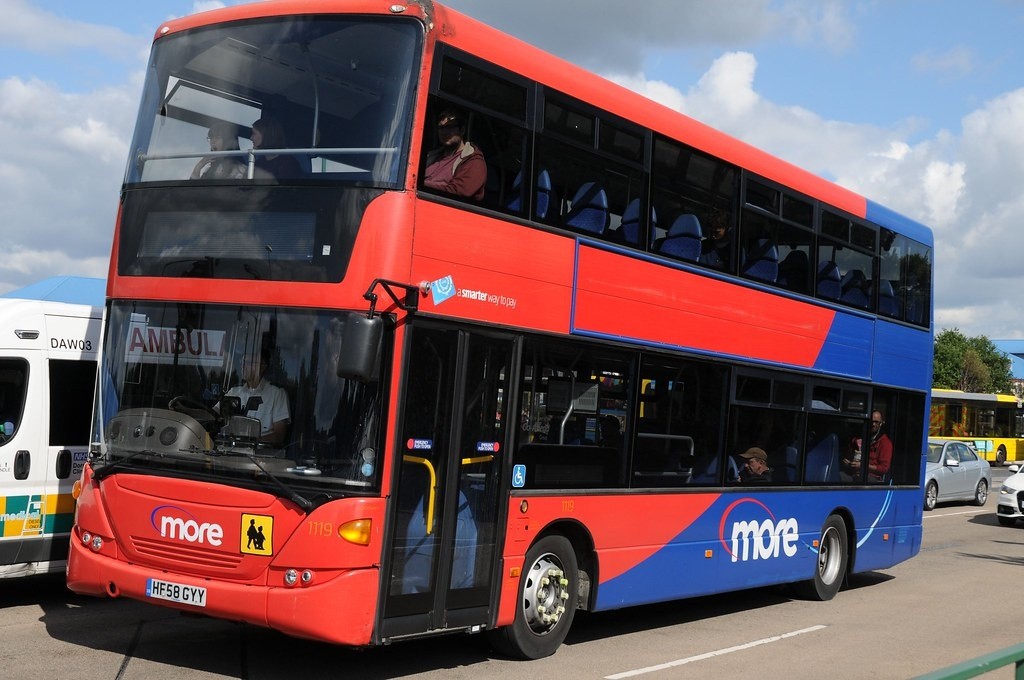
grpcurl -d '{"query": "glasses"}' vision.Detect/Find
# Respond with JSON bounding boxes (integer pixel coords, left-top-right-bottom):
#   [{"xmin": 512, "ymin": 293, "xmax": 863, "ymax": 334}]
[
  {"xmin": 238, "ymin": 358, "xmax": 261, "ymax": 367},
  {"xmin": 437, "ymin": 122, "xmax": 459, "ymax": 131}
]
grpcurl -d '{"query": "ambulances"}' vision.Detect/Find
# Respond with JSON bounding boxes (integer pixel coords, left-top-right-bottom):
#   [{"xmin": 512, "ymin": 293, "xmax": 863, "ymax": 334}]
[{"xmin": 0, "ymin": 298, "xmax": 228, "ymax": 582}]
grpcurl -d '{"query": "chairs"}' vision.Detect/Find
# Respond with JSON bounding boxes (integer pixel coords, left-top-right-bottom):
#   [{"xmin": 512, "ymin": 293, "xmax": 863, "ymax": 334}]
[
  {"xmin": 680, "ymin": 432, "xmax": 840, "ymax": 489},
  {"xmin": 501, "ymin": 157, "xmax": 925, "ymax": 330}
]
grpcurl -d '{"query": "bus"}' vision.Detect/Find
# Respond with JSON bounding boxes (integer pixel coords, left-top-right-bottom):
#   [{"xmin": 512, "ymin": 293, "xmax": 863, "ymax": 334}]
[
  {"xmin": 927, "ymin": 388, "xmax": 1024, "ymax": 468},
  {"xmin": 65, "ymin": 0, "xmax": 936, "ymax": 662}
]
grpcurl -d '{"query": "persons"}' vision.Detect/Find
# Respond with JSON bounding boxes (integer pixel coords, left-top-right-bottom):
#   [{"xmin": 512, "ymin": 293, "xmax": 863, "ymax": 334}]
[
  {"xmin": 189, "ymin": 124, "xmax": 247, "ymax": 179},
  {"xmin": 736, "ymin": 447, "xmax": 777, "ymax": 484},
  {"xmin": 698, "ymin": 209, "xmax": 747, "ymax": 273},
  {"xmin": 212, "ymin": 351, "xmax": 292, "ymax": 449},
  {"xmin": 250, "ymin": 117, "xmax": 304, "ymax": 177},
  {"xmin": 839, "ymin": 410, "xmax": 894, "ymax": 482},
  {"xmin": 422, "ymin": 109, "xmax": 486, "ymax": 199}
]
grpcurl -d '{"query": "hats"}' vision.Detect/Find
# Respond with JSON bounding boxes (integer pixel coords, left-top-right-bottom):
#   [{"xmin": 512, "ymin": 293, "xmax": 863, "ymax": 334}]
[
  {"xmin": 739, "ymin": 447, "xmax": 767, "ymax": 461},
  {"xmin": 598, "ymin": 415, "xmax": 621, "ymax": 430}
]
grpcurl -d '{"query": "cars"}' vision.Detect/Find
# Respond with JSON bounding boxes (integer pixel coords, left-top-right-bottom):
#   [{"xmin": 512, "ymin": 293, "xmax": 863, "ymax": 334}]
[
  {"xmin": 995, "ymin": 462, "xmax": 1024, "ymax": 527},
  {"xmin": 923, "ymin": 439, "xmax": 992, "ymax": 511}
]
